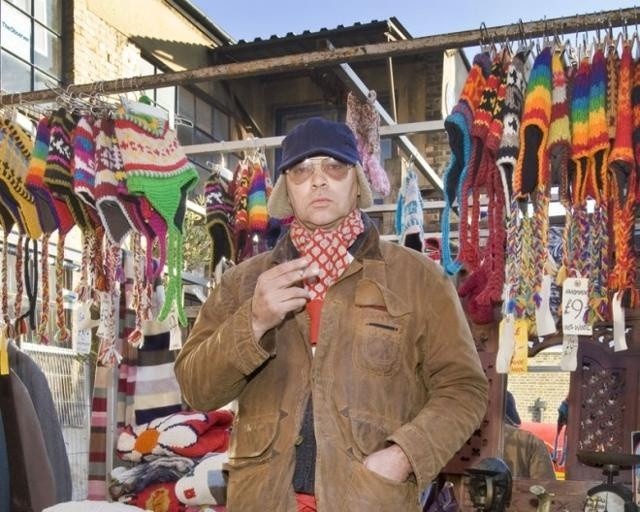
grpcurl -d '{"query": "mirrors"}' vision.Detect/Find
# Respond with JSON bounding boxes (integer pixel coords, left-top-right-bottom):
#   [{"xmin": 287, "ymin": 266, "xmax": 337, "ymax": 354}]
[{"xmin": 440, "ymin": 335, "xmax": 640, "ymax": 481}]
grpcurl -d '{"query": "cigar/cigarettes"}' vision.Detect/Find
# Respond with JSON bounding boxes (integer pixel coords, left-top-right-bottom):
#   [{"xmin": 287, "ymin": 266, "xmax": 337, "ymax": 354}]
[{"xmin": 299, "ymin": 267, "xmax": 304, "ymax": 278}]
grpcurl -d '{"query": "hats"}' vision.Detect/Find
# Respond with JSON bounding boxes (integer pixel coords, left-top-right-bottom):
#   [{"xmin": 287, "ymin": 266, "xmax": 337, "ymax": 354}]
[
  {"xmin": 457, "ymin": 50, "xmax": 503, "ymax": 268},
  {"xmin": 72, "ymin": 115, "xmax": 125, "ymax": 368},
  {"xmin": 266, "ymin": 117, "xmax": 374, "ymax": 219},
  {"xmin": 607, "ymin": 46, "xmax": 637, "ymax": 291},
  {"xmin": 232, "ymin": 166, "xmax": 250, "ymax": 267},
  {"xmin": 631, "ymin": 57, "xmax": 640, "ymax": 214},
  {"xmin": 394, "ymin": 171, "xmax": 426, "ymax": 253},
  {"xmin": 552, "ymin": 395, "xmax": 571, "ymax": 467},
  {"xmin": 112, "ymin": 133, "xmax": 169, "ymax": 284},
  {"xmin": 504, "ymin": 391, "xmax": 522, "ymax": 426},
  {"xmin": 203, "ymin": 172, "xmax": 237, "ymax": 280},
  {"xmin": 500, "ymin": 45, "xmax": 553, "ymax": 322},
  {"xmin": 495, "ymin": 45, "xmax": 534, "ymax": 318},
  {"xmin": 23, "ymin": 117, "xmax": 77, "ymax": 345},
  {"xmin": 248, "ymin": 162, "xmax": 267, "ymax": 257},
  {"xmin": 0, "ymin": 116, "xmax": 44, "ymax": 335},
  {"xmin": 0, "ymin": 193, "xmax": 29, "ymax": 338},
  {"xmin": 566, "ymin": 56, "xmax": 592, "ymax": 280},
  {"xmin": 475, "ymin": 59, "xmax": 513, "ymax": 308},
  {"xmin": 43, "ymin": 106, "xmax": 101, "ymax": 304},
  {"xmin": 582, "ymin": 49, "xmax": 612, "ymax": 325},
  {"xmin": 113, "ymin": 95, "xmax": 201, "ymax": 328},
  {"xmin": 539, "ymin": 54, "xmax": 572, "ymax": 286},
  {"xmin": 440, "ymin": 50, "xmax": 492, "ymax": 275},
  {"xmin": 92, "ymin": 118, "xmax": 154, "ymax": 349},
  {"xmin": 607, "ymin": 46, "xmax": 621, "ymax": 143}
]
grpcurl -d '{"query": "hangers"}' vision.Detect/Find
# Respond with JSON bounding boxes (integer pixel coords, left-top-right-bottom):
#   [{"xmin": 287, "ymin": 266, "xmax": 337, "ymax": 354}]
[
  {"xmin": 1, "ymin": 76, "xmax": 196, "ymax": 129},
  {"xmin": 477, "ymin": 6, "xmax": 640, "ymax": 58},
  {"xmin": 403, "ymin": 143, "xmax": 418, "ymax": 169},
  {"xmin": 206, "ymin": 135, "xmax": 266, "ymax": 179}
]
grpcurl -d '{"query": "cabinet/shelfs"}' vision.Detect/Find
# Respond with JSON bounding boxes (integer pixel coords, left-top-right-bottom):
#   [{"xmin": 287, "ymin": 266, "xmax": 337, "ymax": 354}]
[{"xmin": 1, "ymin": 6, "xmax": 640, "ymax": 512}]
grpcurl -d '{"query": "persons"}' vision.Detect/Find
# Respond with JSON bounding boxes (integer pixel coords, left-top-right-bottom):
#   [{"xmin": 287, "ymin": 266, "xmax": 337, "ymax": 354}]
[{"xmin": 173, "ymin": 117, "xmax": 491, "ymax": 512}]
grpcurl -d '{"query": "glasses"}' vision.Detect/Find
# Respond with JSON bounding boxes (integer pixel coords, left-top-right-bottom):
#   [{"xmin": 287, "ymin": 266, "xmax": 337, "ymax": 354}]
[{"xmin": 286, "ymin": 155, "xmax": 348, "ymax": 185}]
